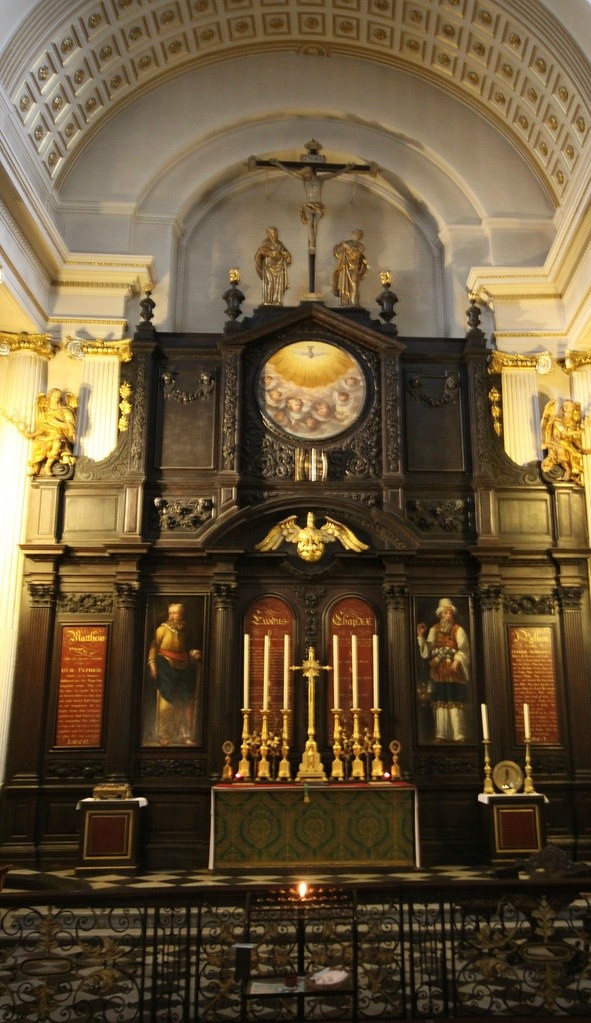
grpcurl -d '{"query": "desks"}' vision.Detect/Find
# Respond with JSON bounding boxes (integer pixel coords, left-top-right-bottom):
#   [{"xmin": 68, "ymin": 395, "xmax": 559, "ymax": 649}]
[
  {"xmin": 210, "ymin": 782, "xmax": 421, "ymax": 874},
  {"xmin": 477, "ymin": 792, "xmax": 549, "ymax": 868},
  {"xmin": 76, "ymin": 798, "xmax": 147, "ymax": 875}
]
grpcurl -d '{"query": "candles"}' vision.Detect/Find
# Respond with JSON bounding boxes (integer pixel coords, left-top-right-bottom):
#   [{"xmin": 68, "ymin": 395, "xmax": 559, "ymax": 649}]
[
  {"xmin": 481, "ymin": 704, "xmax": 490, "ymax": 740},
  {"xmin": 282, "ymin": 634, "xmax": 290, "ymax": 709},
  {"xmin": 373, "ymin": 634, "xmax": 382, "ymax": 708},
  {"xmin": 332, "ymin": 634, "xmax": 340, "ymax": 708},
  {"xmin": 352, "ymin": 634, "xmax": 359, "ymax": 708},
  {"xmin": 243, "ymin": 634, "xmax": 251, "ymax": 708},
  {"xmin": 523, "ymin": 703, "xmax": 531, "ymax": 738},
  {"xmin": 262, "ymin": 635, "xmax": 270, "ymax": 710}
]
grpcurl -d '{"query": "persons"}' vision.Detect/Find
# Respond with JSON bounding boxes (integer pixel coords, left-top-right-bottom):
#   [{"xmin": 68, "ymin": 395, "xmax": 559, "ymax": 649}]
[
  {"xmin": 269, "ymin": 158, "xmax": 355, "ymax": 255},
  {"xmin": 28, "ymin": 388, "xmax": 78, "ymax": 475},
  {"xmin": 332, "ymin": 229, "xmax": 370, "ymax": 307},
  {"xmin": 255, "ymin": 224, "xmax": 292, "ymax": 307},
  {"xmin": 554, "ymin": 399, "xmax": 591, "ymax": 486}
]
